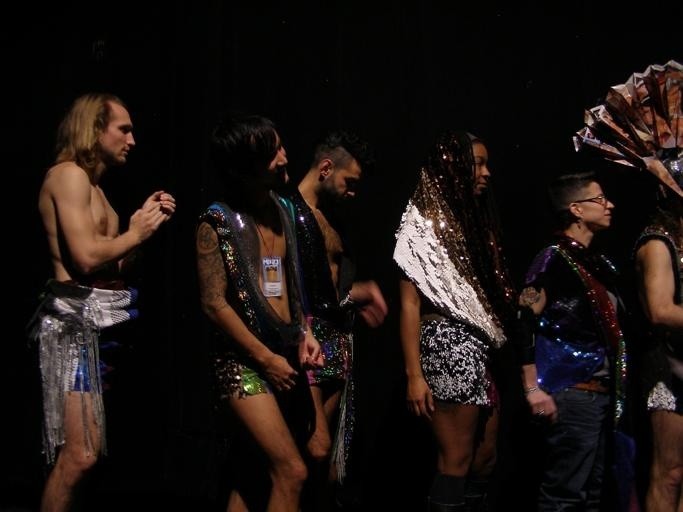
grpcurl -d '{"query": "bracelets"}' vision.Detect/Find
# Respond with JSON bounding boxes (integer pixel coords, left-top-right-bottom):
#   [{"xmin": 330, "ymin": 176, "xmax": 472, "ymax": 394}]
[{"xmin": 523, "ymin": 386, "xmax": 540, "ymax": 397}]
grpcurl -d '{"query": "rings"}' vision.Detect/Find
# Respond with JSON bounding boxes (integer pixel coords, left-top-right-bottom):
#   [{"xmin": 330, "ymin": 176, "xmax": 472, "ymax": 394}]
[{"xmin": 538, "ymin": 407, "xmax": 544, "ymax": 417}]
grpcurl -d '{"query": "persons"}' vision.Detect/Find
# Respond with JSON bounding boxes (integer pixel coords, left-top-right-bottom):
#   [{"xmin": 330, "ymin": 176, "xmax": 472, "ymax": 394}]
[
  {"xmin": 28, "ymin": 90, "xmax": 176, "ymax": 512},
  {"xmin": 277, "ymin": 129, "xmax": 391, "ymax": 512},
  {"xmin": 195, "ymin": 114, "xmax": 350, "ymax": 512},
  {"xmin": 392, "ymin": 129, "xmax": 549, "ymax": 512},
  {"xmin": 515, "ymin": 172, "xmax": 633, "ymax": 510},
  {"xmin": 630, "ymin": 143, "xmax": 683, "ymax": 511}
]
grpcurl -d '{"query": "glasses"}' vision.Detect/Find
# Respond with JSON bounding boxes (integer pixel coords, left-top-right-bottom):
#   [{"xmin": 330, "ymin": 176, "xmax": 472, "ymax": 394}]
[{"xmin": 574, "ymin": 195, "xmax": 608, "ymax": 205}]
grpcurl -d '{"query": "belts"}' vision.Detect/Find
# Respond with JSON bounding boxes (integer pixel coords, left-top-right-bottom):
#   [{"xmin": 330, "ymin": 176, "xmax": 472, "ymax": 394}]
[{"xmin": 576, "ymin": 383, "xmax": 610, "ymax": 393}]
